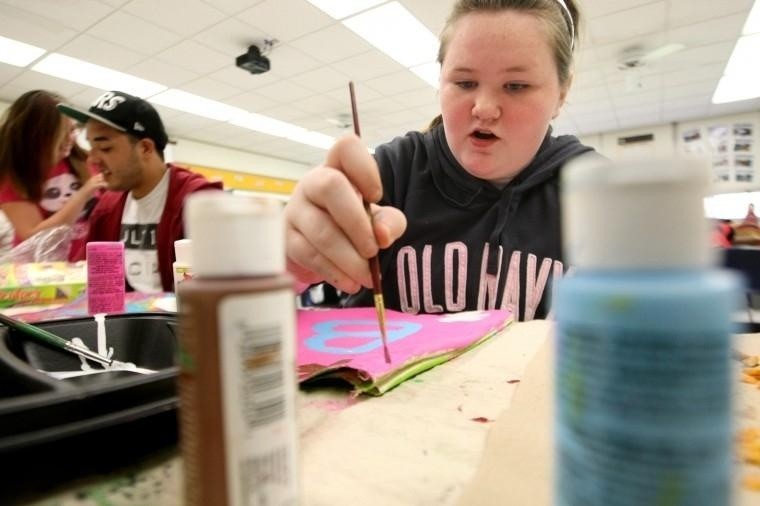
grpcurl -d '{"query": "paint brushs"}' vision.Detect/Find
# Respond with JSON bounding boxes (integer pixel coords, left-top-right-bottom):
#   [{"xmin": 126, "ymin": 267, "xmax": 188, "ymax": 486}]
[{"xmin": 350, "ymin": 83, "xmax": 389, "ymax": 362}]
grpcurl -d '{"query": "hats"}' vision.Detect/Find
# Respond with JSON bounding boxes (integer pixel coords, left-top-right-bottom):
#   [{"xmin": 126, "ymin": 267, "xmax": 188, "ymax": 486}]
[{"xmin": 56, "ymin": 91, "xmax": 167, "ymax": 146}]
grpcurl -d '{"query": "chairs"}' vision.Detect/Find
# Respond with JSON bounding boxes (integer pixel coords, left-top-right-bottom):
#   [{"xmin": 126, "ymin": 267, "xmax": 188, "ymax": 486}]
[{"xmin": 726, "ymin": 249, "xmax": 760, "ymax": 308}]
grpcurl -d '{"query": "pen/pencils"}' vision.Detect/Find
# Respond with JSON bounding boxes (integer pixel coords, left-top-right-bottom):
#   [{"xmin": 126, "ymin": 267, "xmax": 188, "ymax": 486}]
[{"xmin": 1, "ymin": 314, "xmax": 115, "ymax": 366}]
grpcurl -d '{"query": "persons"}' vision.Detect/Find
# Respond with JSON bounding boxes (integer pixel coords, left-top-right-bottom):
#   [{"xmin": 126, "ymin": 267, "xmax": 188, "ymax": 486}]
[
  {"xmin": 283, "ymin": 0, "xmax": 613, "ymax": 323},
  {"xmin": 57, "ymin": 90, "xmax": 226, "ymax": 294},
  {"xmin": 0, "ymin": 89, "xmax": 108, "ymax": 265},
  {"xmin": 709, "ymin": 203, "xmax": 760, "ymax": 253}
]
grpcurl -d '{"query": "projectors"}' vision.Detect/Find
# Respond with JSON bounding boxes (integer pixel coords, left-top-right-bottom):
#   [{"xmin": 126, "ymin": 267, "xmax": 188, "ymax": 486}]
[{"xmin": 235, "ymin": 46, "xmax": 270, "ymax": 74}]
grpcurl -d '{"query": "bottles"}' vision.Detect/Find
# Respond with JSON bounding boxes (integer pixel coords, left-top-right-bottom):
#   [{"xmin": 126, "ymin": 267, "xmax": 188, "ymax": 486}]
[
  {"xmin": 546, "ymin": 137, "xmax": 746, "ymax": 505},
  {"xmin": 171, "ymin": 185, "xmax": 305, "ymax": 505}
]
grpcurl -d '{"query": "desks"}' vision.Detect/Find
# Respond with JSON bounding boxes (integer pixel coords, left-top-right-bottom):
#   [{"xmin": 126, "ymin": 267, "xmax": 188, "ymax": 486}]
[{"xmin": 6, "ymin": 318, "xmax": 760, "ymax": 506}]
